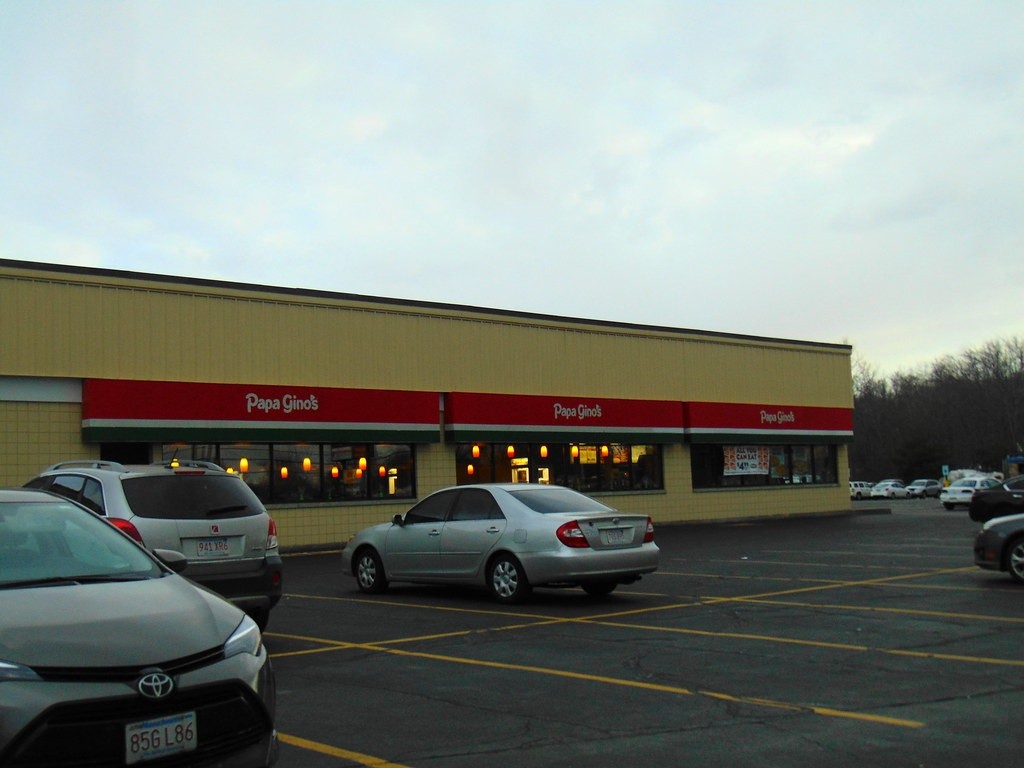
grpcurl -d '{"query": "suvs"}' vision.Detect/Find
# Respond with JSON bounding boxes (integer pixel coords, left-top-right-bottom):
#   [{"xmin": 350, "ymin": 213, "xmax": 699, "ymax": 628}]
[{"xmin": 17, "ymin": 459, "xmax": 287, "ymax": 636}]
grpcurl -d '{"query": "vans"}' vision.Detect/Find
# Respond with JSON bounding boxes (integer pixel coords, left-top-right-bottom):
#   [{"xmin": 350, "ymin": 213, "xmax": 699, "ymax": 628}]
[{"xmin": 849, "ymin": 481, "xmax": 872, "ymax": 501}]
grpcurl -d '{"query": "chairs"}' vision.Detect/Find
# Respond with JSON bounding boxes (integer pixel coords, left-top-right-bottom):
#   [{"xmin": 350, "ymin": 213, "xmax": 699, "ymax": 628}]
[{"xmin": 170, "ymin": 457, "xmax": 387, "ymax": 482}]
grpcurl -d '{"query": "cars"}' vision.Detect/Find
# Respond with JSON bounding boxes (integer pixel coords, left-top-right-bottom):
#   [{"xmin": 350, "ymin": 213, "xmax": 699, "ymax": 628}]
[
  {"xmin": 782, "ymin": 472, "xmax": 822, "ymax": 485},
  {"xmin": 342, "ymin": 481, "xmax": 661, "ymax": 606},
  {"xmin": 869, "ymin": 479, "xmax": 904, "ymax": 488},
  {"xmin": 974, "ymin": 512, "xmax": 1024, "ymax": 585},
  {"xmin": 0, "ymin": 486, "xmax": 273, "ymax": 768},
  {"xmin": 905, "ymin": 479, "xmax": 943, "ymax": 500},
  {"xmin": 870, "ymin": 481, "xmax": 912, "ymax": 500},
  {"xmin": 969, "ymin": 473, "xmax": 1024, "ymax": 524},
  {"xmin": 940, "ymin": 476, "xmax": 1010, "ymax": 511},
  {"xmin": 944, "ymin": 470, "xmax": 980, "ymax": 489}
]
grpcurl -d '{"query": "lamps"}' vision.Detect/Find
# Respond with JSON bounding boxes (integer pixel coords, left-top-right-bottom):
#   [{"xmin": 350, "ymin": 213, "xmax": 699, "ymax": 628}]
[
  {"xmin": 601, "ymin": 445, "xmax": 608, "ymax": 457},
  {"xmin": 541, "ymin": 446, "xmax": 548, "ymax": 457},
  {"xmin": 571, "ymin": 446, "xmax": 579, "ymax": 457},
  {"xmin": 507, "ymin": 446, "xmax": 514, "ymax": 458},
  {"xmin": 473, "ymin": 445, "xmax": 480, "ymax": 458},
  {"xmin": 468, "ymin": 464, "xmax": 475, "ymax": 473}
]
